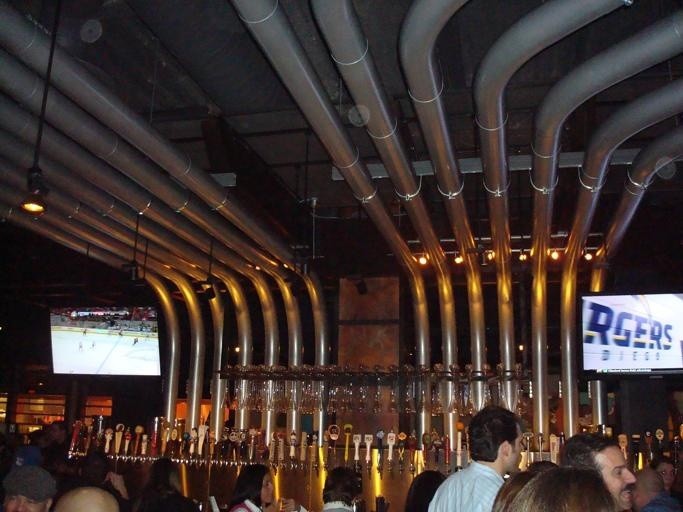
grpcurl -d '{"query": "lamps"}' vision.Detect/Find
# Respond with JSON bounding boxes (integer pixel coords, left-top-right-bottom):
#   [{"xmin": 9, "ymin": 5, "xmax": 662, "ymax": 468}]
[
  {"xmin": 413, "ymin": 237, "xmax": 597, "ymax": 266},
  {"xmin": 199, "ymin": 234, "xmax": 220, "ymax": 302},
  {"xmin": 17, "ymin": 2, "xmax": 65, "ymax": 216},
  {"xmin": 126, "ymin": 208, "xmax": 140, "ymax": 286}
]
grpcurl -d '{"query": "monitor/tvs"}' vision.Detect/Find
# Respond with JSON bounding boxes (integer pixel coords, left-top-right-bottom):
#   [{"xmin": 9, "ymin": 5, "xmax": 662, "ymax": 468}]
[
  {"xmin": 44, "ymin": 297, "xmax": 162, "ymax": 379},
  {"xmin": 575, "ymin": 291, "xmax": 682, "ymax": 381}
]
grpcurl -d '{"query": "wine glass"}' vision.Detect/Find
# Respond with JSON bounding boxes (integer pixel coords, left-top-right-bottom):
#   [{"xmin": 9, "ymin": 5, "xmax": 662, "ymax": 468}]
[{"xmin": 215, "ymin": 363, "xmax": 530, "ymax": 416}]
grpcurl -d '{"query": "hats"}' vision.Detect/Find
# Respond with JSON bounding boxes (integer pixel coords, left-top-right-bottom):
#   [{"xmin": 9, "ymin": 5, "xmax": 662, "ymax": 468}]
[{"xmin": 2, "ymin": 464, "xmax": 57, "ymax": 500}]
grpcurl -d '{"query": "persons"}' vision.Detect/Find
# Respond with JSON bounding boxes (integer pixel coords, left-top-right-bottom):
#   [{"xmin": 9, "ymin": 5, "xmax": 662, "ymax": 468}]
[
  {"xmin": 51, "ymin": 311, "xmax": 158, "ymax": 346},
  {"xmin": 0, "ymin": 404, "xmax": 683, "ymax": 511}
]
